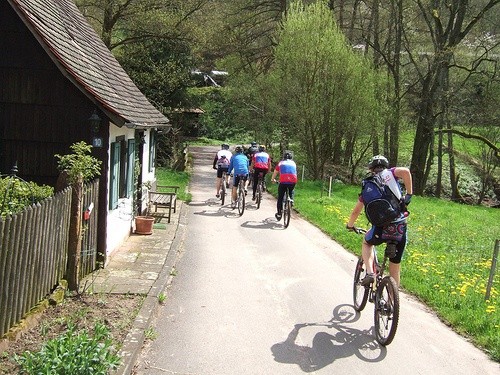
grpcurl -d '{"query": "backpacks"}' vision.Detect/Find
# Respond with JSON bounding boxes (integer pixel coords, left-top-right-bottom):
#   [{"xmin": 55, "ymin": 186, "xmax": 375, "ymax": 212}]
[{"xmin": 361, "ymin": 171, "xmax": 404, "ymax": 227}]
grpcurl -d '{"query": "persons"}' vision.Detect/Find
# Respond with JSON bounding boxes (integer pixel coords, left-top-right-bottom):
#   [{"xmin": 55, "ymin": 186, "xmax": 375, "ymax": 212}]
[
  {"xmin": 272, "ymin": 150, "xmax": 298, "ymax": 221},
  {"xmin": 346, "ymin": 154, "xmax": 413, "ymax": 314},
  {"xmin": 213, "ymin": 141, "xmax": 271, "ymax": 201}
]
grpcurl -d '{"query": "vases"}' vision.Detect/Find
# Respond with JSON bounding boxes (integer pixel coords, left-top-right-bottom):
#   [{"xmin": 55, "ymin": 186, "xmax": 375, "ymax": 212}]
[{"xmin": 134, "ymin": 216, "xmax": 155, "ymax": 232}]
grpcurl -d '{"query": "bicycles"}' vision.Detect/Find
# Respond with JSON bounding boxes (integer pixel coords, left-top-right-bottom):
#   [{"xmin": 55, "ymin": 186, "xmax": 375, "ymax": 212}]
[
  {"xmin": 271, "ymin": 178, "xmax": 294, "ymax": 228},
  {"xmin": 347, "ymin": 226, "xmax": 400, "ymax": 346},
  {"xmin": 214, "ymin": 161, "xmax": 266, "ymax": 210},
  {"xmin": 227, "ymin": 173, "xmax": 248, "ymax": 216}
]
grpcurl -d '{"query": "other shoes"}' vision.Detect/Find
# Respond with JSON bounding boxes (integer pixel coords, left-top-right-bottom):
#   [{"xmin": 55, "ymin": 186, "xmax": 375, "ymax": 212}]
[
  {"xmin": 385, "ymin": 302, "xmax": 396, "ymax": 312},
  {"xmin": 262, "ymin": 184, "xmax": 266, "ymax": 192},
  {"xmin": 291, "ymin": 200, "xmax": 294, "ymax": 206},
  {"xmin": 226, "ymin": 182, "xmax": 230, "ymax": 188},
  {"xmin": 361, "ymin": 272, "xmax": 374, "ymax": 286},
  {"xmin": 275, "ymin": 213, "xmax": 282, "ymax": 221},
  {"xmin": 231, "ymin": 201, "xmax": 236, "ymax": 209},
  {"xmin": 244, "ymin": 187, "xmax": 248, "ymax": 196},
  {"xmin": 252, "ymin": 197, "xmax": 256, "ymax": 201},
  {"xmin": 215, "ymin": 194, "xmax": 220, "ymax": 198}
]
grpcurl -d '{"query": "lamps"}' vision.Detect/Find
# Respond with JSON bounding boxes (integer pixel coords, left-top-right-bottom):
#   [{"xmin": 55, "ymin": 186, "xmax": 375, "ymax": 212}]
[{"xmin": 88, "ymin": 110, "xmax": 102, "ymax": 133}]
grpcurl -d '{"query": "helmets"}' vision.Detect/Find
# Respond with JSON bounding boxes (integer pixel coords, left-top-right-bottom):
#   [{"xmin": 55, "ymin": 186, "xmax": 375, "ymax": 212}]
[
  {"xmin": 369, "ymin": 155, "xmax": 389, "ymax": 169},
  {"xmin": 251, "ymin": 141, "xmax": 256, "ymax": 146},
  {"xmin": 258, "ymin": 145, "xmax": 266, "ymax": 150},
  {"xmin": 235, "ymin": 147, "xmax": 243, "ymax": 152},
  {"xmin": 284, "ymin": 152, "xmax": 292, "ymax": 159},
  {"xmin": 221, "ymin": 143, "xmax": 229, "ymax": 149}
]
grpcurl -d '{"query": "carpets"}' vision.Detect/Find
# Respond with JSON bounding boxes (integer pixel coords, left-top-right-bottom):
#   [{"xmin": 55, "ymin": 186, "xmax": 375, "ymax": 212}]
[{"xmin": 153, "ymin": 223, "xmax": 166, "ymax": 229}]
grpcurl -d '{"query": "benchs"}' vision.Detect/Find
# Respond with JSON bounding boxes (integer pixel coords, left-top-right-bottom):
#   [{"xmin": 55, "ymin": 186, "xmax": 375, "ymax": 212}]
[{"xmin": 148, "ymin": 181, "xmax": 180, "ymax": 223}]
